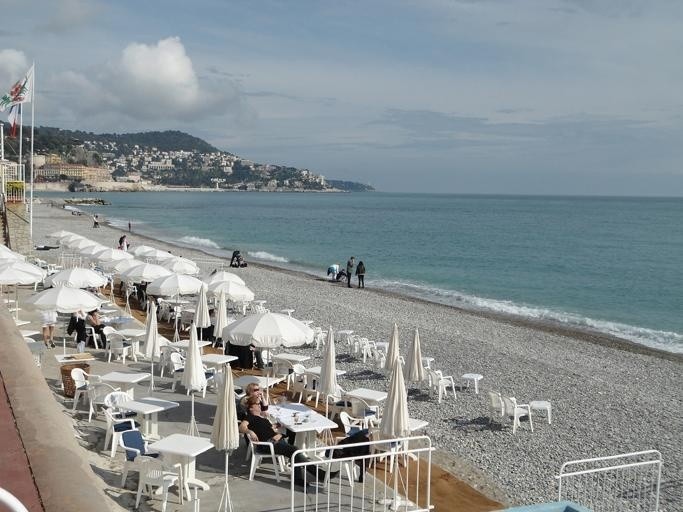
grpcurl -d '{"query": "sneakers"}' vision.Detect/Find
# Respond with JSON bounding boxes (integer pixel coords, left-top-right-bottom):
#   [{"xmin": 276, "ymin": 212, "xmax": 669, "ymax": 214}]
[{"xmin": 331, "ymin": 470, "xmax": 339, "ymax": 480}]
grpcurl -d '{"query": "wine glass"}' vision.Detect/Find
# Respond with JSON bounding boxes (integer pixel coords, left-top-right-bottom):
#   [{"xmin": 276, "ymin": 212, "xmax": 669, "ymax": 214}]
[{"xmin": 271, "ymin": 397, "xmax": 310, "ymax": 426}]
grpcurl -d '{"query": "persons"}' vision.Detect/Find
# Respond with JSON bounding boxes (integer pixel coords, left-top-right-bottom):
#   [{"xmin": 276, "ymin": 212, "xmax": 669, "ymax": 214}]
[
  {"xmin": 94, "ymin": 216, "xmax": 99, "ymax": 228},
  {"xmin": 328, "ymin": 256, "xmax": 366, "ymax": 289},
  {"xmin": 237, "ymin": 383, "xmax": 340, "ymax": 487}
]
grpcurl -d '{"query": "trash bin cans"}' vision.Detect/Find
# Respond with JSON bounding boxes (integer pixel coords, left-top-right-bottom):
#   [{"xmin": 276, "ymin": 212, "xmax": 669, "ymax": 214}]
[{"xmin": 61, "ymin": 364, "xmax": 90, "ymax": 398}]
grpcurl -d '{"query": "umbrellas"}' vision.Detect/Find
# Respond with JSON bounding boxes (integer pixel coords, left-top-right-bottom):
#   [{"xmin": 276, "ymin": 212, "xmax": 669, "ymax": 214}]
[
  {"xmin": 209, "ymin": 363, "xmax": 240, "ymax": 512},
  {"xmin": 403, "ymin": 327, "xmax": 425, "ymax": 393},
  {"xmin": 315, "ymin": 326, "xmax": 342, "ymax": 418},
  {"xmin": 143, "ymin": 300, "xmax": 160, "ymax": 390},
  {"xmin": 0, "ymin": 228, "xmax": 255, "ymax": 354},
  {"xmin": 385, "ymin": 323, "xmax": 400, "ymax": 379},
  {"xmin": 222, "ymin": 310, "xmax": 314, "ymax": 403},
  {"xmin": 181, "ymin": 322, "xmax": 206, "ymax": 437},
  {"xmin": 379, "ymin": 355, "xmax": 412, "ymax": 497}
]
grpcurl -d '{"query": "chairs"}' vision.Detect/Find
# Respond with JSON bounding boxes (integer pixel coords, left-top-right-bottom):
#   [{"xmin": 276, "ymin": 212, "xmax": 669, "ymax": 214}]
[
  {"xmin": 48, "ymin": 263, "xmax": 242, "ymax": 508},
  {"xmin": 180, "ymin": 263, "xmax": 552, "ymax": 435},
  {"xmin": 229, "ymin": 349, "xmax": 433, "ymax": 494}
]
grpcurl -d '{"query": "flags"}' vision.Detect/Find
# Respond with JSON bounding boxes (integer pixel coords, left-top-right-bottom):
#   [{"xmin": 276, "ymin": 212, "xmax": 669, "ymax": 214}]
[
  {"xmin": 0, "ymin": 66, "xmax": 32, "ymax": 111},
  {"xmin": 7, "ymin": 104, "xmax": 19, "ymax": 138}
]
